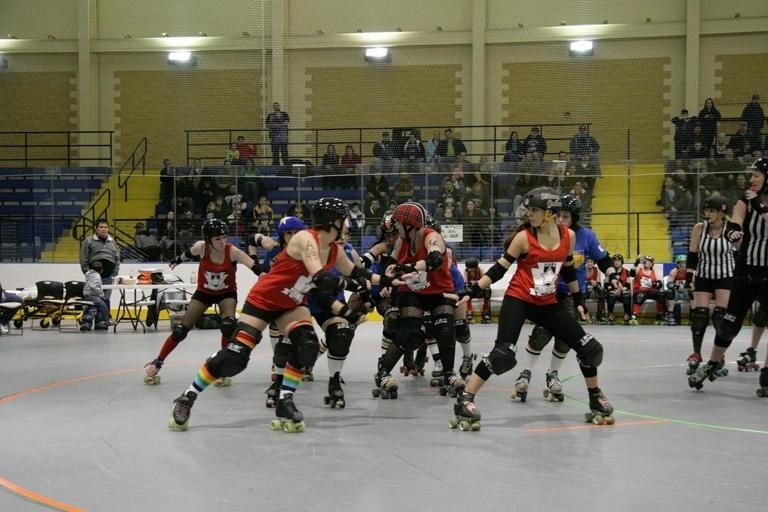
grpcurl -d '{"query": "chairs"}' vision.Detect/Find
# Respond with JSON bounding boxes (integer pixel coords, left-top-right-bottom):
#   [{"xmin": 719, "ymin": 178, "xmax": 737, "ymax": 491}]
[
  {"xmin": 1, "ymin": 277, "xmax": 200, "ymax": 332},
  {"xmin": 454, "ymin": 264, "xmax": 689, "ymax": 325}
]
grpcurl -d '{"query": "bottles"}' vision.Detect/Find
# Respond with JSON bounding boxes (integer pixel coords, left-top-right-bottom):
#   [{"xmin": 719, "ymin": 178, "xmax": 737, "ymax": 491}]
[{"xmin": 190, "ymin": 271, "xmax": 196, "ymax": 284}]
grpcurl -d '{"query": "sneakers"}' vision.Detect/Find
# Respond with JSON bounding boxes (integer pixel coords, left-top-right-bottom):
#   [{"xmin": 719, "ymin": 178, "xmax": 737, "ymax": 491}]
[
  {"xmin": 95, "ymin": 323, "xmax": 109, "ymax": 331},
  {"xmin": 0, "ymin": 324, "xmax": 10, "ymax": 334},
  {"xmin": 80, "ymin": 325, "xmax": 90, "ymax": 331}
]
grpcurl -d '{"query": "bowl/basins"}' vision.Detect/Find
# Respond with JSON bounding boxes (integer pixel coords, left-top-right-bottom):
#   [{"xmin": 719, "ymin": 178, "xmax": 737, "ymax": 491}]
[{"xmin": 111, "ymin": 276, "xmax": 137, "ymax": 285}]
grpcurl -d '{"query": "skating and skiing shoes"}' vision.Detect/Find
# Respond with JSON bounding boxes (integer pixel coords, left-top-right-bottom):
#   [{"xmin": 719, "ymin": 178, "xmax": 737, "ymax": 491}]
[
  {"xmin": 301, "ymin": 366, "xmax": 314, "ymax": 381},
  {"xmin": 595, "ymin": 313, "xmax": 677, "ymax": 326},
  {"xmin": 578, "ymin": 314, "xmax": 587, "ymax": 324},
  {"xmin": 480, "ymin": 313, "xmax": 490, "ymax": 323},
  {"xmin": 167, "ymin": 389, "xmax": 197, "ymax": 430},
  {"xmin": 144, "ymin": 361, "xmax": 162, "ymax": 384},
  {"xmin": 322, "ymin": 371, "xmax": 346, "ymax": 408},
  {"xmin": 510, "ymin": 370, "xmax": 531, "ymax": 402},
  {"xmin": 466, "ymin": 311, "xmax": 472, "ymax": 322},
  {"xmin": 372, "ymin": 351, "xmax": 479, "ymax": 400},
  {"xmin": 264, "ymin": 374, "xmax": 286, "ymax": 407},
  {"xmin": 448, "ymin": 391, "xmax": 481, "ymax": 431},
  {"xmin": 686, "ymin": 348, "xmax": 767, "ymax": 397},
  {"xmin": 215, "ymin": 376, "xmax": 234, "ymax": 388},
  {"xmin": 271, "ymin": 393, "xmax": 306, "ymax": 433},
  {"xmin": 584, "ymin": 386, "xmax": 615, "ymax": 424},
  {"xmin": 542, "ymin": 370, "xmax": 564, "ymax": 402}
]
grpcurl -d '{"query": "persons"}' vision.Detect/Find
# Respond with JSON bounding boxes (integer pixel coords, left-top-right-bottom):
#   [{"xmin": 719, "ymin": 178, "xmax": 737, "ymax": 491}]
[
  {"xmin": 79, "ymin": 217, "xmax": 120, "ymax": 333},
  {"xmin": 651, "ymin": 90, "xmax": 768, "ymax": 220},
  {"xmin": 629, "ymin": 254, "xmax": 670, "ymax": 321},
  {"xmin": 133, "ymin": 98, "xmax": 600, "ymax": 249},
  {"xmin": 662, "ymin": 253, "xmax": 693, "ymax": 325},
  {"xmin": 81, "ymin": 261, "xmax": 118, "ymax": 327},
  {"xmin": 140, "ymin": 217, "xmax": 268, "ymax": 380},
  {"xmin": 172, "ymin": 186, "xmax": 631, "ymax": 433},
  {"xmin": 0, "ymin": 285, "xmax": 24, "ymax": 335},
  {"xmin": 686, "ymin": 156, "xmax": 768, "ymax": 388},
  {"xmin": 757, "ymin": 351, "xmax": 768, "ymax": 388},
  {"xmin": 683, "ymin": 194, "xmax": 738, "ymax": 371},
  {"xmin": 736, "ymin": 302, "xmax": 768, "ymax": 364}
]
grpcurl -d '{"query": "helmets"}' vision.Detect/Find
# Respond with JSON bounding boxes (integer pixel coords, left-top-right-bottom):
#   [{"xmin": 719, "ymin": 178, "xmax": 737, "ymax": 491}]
[
  {"xmin": 277, "ymin": 198, "xmax": 441, "ymax": 242},
  {"xmin": 643, "ymin": 256, "xmax": 654, "ymax": 266},
  {"xmin": 676, "ymin": 254, "xmax": 687, "ymax": 263},
  {"xmin": 201, "ymin": 219, "xmax": 229, "ymax": 245},
  {"xmin": 523, "ymin": 187, "xmax": 564, "ymax": 215},
  {"xmin": 612, "ymin": 253, "xmax": 624, "ymax": 266},
  {"xmin": 700, "ymin": 195, "xmax": 729, "ymax": 214},
  {"xmin": 750, "ymin": 157, "xmax": 768, "ymax": 176},
  {"xmin": 465, "ymin": 257, "xmax": 478, "ymax": 269},
  {"xmin": 559, "ymin": 193, "xmax": 582, "ymax": 213}
]
